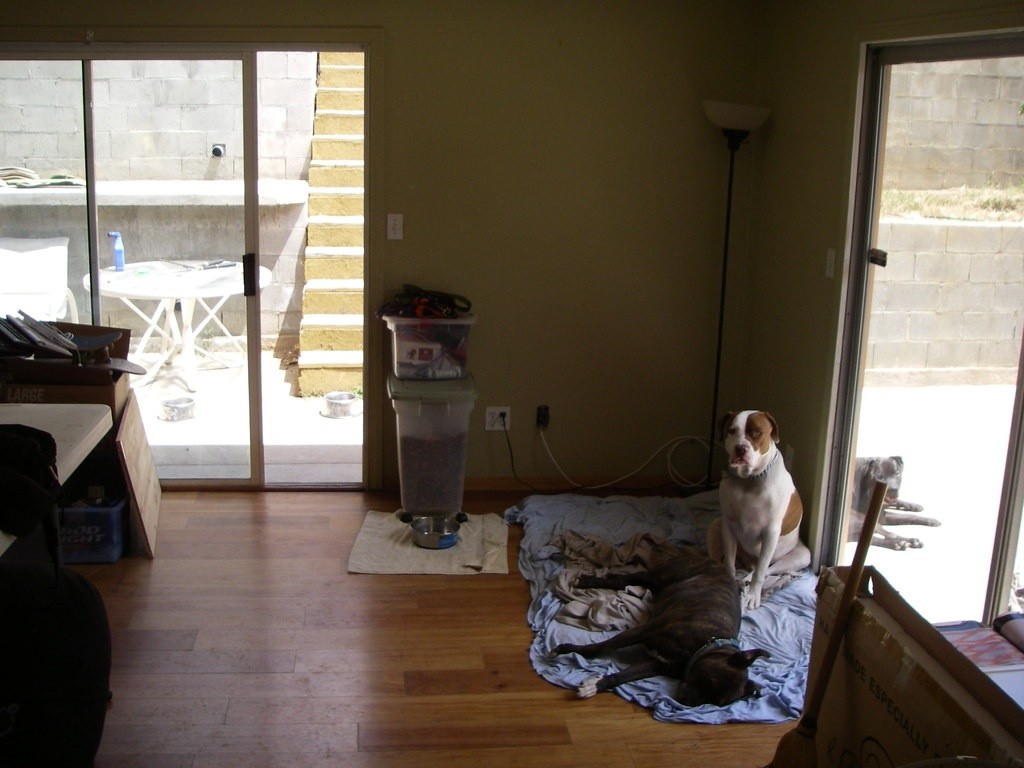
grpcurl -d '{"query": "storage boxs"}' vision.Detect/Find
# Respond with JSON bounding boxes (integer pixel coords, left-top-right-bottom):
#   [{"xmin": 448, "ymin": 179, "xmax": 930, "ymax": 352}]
[
  {"xmin": 0, "ymin": 311, "xmax": 140, "ymax": 564},
  {"xmin": 383, "ymin": 313, "xmax": 480, "ymax": 380},
  {"xmin": 797, "ymin": 566, "xmax": 1024, "ymax": 768},
  {"xmin": 386, "ymin": 369, "xmax": 478, "ymax": 516}
]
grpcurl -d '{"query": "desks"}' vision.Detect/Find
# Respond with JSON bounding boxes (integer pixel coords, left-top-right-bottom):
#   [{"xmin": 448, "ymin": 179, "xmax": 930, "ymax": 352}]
[
  {"xmin": 0, "ymin": 402, "xmax": 115, "ymax": 562},
  {"xmin": 82, "ymin": 258, "xmax": 274, "ymax": 389}
]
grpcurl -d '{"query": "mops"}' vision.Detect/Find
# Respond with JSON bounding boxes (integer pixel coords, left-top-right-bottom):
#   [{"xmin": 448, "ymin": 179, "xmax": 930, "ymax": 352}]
[{"xmin": 769, "ymin": 479, "xmax": 890, "ymax": 768}]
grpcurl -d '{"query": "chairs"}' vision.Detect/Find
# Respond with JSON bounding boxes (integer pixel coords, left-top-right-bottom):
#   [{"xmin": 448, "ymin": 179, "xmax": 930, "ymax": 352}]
[{"xmin": 0, "ymin": 236, "xmax": 79, "ymax": 322}]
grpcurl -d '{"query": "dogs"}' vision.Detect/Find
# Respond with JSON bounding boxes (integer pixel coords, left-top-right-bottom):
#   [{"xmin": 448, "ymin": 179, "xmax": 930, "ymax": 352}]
[
  {"xmin": 553, "ymin": 551, "xmax": 772, "ymax": 707},
  {"xmin": 846, "ymin": 455, "xmax": 942, "ymax": 551},
  {"xmin": 705, "ymin": 410, "xmax": 811, "ymax": 608}
]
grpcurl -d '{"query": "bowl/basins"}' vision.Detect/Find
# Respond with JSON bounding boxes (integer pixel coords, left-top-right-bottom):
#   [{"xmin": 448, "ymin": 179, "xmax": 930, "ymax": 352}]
[
  {"xmin": 157, "ymin": 399, "xmax": 195, "ymax": 422},
  {"xmin": 410, "ymin": 516, "xmax": 461, "ymax": 549},
  {"xmin": 322, "ymin": 391, "xmax": 360, "ymax": 418}
]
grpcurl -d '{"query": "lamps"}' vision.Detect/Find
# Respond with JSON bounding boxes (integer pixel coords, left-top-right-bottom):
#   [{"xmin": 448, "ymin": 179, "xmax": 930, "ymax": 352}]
[{"xmin": 682, "ymin": 98, "xmax": 774, "ymax": 499}]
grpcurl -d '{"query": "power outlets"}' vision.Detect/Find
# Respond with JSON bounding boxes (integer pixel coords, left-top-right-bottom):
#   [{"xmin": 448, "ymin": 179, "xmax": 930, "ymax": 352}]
[
  {"xmin": 485, "ymin": 407, "xmax": 511, "ymax": 432},
  {"xmin": 537, "ymin": 405, "xmax": 550, "ymax": 429},
  {"xmin": 387, "ymin": 213, "xmax": 403, "ymax": 240}
]
